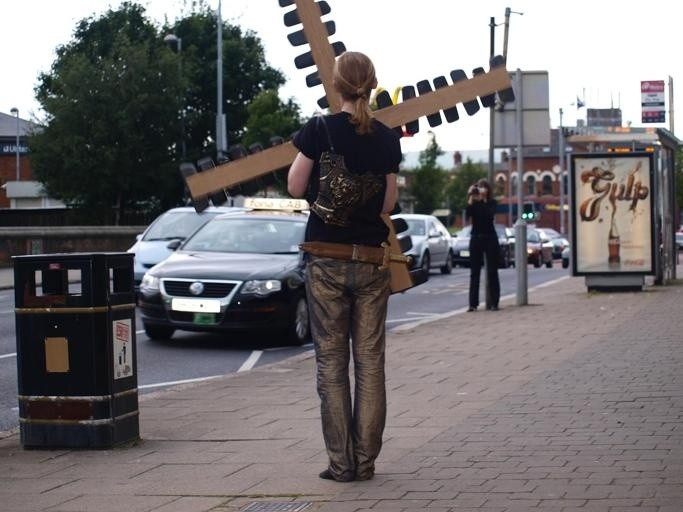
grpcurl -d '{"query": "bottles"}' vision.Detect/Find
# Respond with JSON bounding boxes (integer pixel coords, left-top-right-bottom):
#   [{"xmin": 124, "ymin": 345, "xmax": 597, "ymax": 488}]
[{"xmin": 607, "ymin": 215, "xmax": 621, "ymax": 263}]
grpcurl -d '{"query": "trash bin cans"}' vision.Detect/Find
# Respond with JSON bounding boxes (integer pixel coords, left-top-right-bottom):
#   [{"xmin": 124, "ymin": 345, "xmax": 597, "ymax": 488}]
[{"xmin": 12, "ymin": 252, "xmax": 142, "ymax": 448}]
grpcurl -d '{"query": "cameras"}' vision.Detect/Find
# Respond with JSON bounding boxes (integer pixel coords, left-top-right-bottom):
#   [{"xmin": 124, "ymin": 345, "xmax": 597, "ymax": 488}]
[{"xmin": 471, "ymin": 183, "xmax": 480, "ymax": 195}]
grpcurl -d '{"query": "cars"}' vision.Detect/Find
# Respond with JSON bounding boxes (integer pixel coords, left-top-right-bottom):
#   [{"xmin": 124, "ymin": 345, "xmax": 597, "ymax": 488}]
[
  {"xmin": 456, "ymin": 225, "xmax": 570, "ymax": 269},
  {"xmin": 128, "ymin": 198, "xmax": 312, "ymax": 346},
  {"xmin": 388, "ymin": 213, "xmax": 454, "ymax": 275}
]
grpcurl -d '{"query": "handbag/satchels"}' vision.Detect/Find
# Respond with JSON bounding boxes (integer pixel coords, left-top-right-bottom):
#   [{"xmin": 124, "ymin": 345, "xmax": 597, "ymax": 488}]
[{"xmin": 305, "ymin": 151, "xmax": 386, "ymax": 229}]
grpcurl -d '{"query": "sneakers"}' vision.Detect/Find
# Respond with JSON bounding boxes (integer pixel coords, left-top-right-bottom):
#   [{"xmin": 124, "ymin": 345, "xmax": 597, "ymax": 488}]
[{"xmin": 319, "ymin": 470, "xmax": 335, "ymax": 479}]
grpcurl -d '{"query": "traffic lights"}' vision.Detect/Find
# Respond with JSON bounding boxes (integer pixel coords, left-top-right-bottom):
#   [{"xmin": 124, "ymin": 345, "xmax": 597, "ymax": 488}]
[{"xmin": 521, "ymin": 203, "xmax": 536, "ymax": 221}]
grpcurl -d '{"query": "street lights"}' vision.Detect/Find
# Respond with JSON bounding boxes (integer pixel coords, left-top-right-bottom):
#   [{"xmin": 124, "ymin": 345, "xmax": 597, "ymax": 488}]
[
  {"xmin": 164, "ymin": 34, "xmax": 187, "ymax": 161},
  {"xmin": 11, "ymin": 109, "xmax": 20, "ymax": 178}
]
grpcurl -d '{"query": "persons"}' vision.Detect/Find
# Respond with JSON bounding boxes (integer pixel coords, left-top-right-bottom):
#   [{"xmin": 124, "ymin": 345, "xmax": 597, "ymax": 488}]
[
  {"xmin": 287, "ymin": 52, "xmax": 403, "ymax": 482},
  {"xmin": 465, "ymin": 178, "xmax": 500, "ymax": 312}
]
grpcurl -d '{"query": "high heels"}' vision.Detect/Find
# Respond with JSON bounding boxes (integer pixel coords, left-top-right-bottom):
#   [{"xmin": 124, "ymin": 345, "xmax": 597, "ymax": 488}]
[
  {"xmin": 487, "ymin": 305, "xmax": 499, "ymax": 310},
  {"xmin": 468, "ymin": 306, "xmax": 477, "ymax": 312}
]
